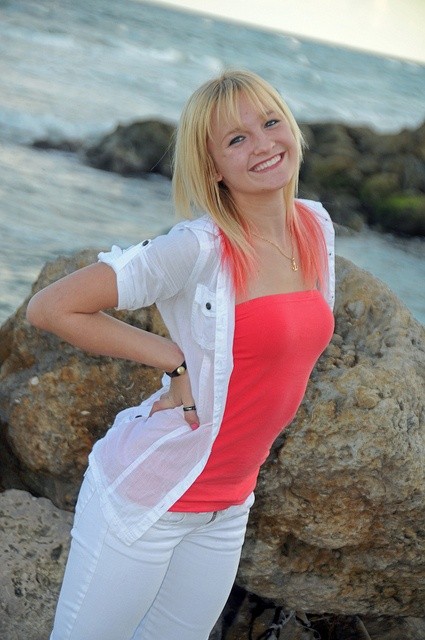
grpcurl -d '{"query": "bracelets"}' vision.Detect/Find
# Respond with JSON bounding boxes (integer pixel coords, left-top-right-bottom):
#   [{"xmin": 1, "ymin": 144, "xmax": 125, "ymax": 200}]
[{"xmin": 165, "ymin": 360, "xmax": 187, "ymax": 377}]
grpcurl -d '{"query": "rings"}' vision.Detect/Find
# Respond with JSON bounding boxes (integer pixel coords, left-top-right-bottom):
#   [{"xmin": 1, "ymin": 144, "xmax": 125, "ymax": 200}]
[{"xmin": 183, "ymin": 406, "xmax": 196, "ymax": 411}]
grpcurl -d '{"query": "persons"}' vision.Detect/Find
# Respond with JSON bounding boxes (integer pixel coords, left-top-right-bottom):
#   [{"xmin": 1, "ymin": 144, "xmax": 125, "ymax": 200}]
[{"xmin": 23, "ymin": 69, "xmax": 339, "ymax": 640}]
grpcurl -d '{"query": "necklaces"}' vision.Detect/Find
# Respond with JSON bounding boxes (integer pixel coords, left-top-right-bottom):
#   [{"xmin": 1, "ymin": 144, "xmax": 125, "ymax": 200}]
[{"xmin": 250, "ymin": 229, "xmax": 299, "ymax": 272}]
